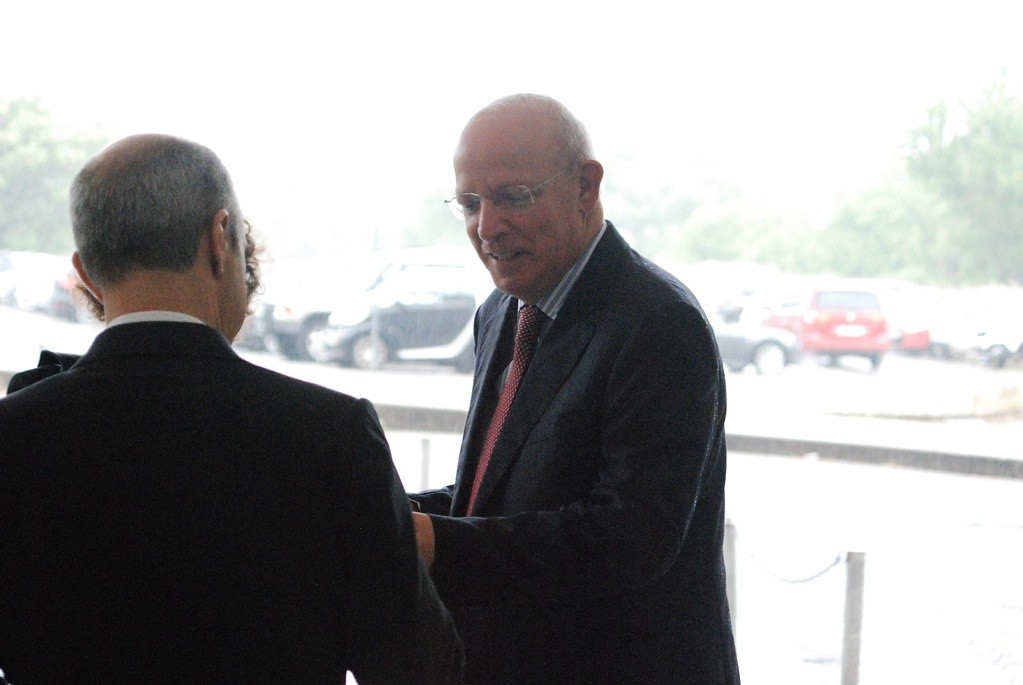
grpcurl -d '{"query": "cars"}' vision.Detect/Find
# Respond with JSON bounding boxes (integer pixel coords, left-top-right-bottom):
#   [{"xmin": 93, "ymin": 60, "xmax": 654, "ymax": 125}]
[
  {"xmin": 0, "ymin": 248, "xmax": 499, "ymax": 376},
  {"xmin": 696, "ymin": 273, "xmax": 1023, "ymax": 376}
]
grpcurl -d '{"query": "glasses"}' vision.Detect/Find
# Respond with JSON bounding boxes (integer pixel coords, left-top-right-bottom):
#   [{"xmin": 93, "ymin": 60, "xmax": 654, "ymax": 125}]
[{"xmin": 443, "ymin": 171, "xmax": 565, "ymax": 213}]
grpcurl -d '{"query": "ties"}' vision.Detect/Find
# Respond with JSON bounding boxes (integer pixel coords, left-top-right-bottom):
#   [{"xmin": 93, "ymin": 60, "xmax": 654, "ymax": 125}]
[{"xmin": 466, "ymin": 306, "xmax": 546, "ymax": 517}]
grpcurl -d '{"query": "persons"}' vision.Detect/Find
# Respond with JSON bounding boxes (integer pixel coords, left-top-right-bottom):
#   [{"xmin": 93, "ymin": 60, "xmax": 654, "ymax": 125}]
[{"xmin": 0, "ymin": 93, "xmax": 740, "ymax": 685}]
